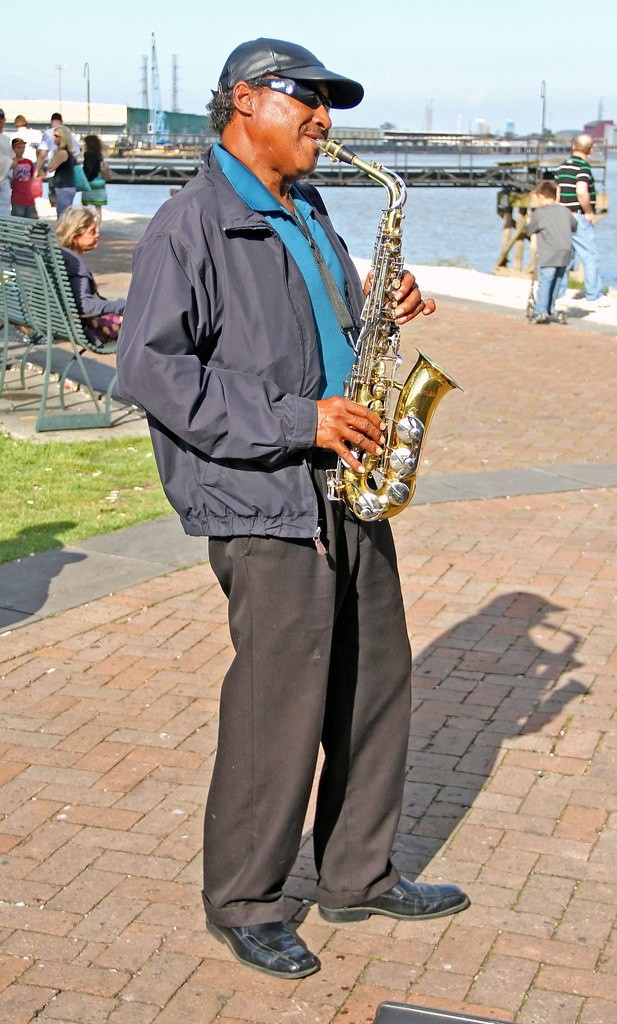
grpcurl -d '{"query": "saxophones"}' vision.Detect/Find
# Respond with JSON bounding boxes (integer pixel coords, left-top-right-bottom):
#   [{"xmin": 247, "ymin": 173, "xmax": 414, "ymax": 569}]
[{"xmin": 312, "ymin": 135, "xmax": 468, "ymax": 522}]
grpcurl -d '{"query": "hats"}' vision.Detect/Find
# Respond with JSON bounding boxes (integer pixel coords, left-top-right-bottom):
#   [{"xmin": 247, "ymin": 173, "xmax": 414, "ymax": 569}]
[
  {"xmin": 220, "ymin": 37, "xmax": 365, "ymax": 110},
  {"xmin": 0, "ymin": 109, "xmax": 4, "ymax": 119},
  {"xmin": 11, "ymin": 138, "xmax": 26, "ymax": 147}
]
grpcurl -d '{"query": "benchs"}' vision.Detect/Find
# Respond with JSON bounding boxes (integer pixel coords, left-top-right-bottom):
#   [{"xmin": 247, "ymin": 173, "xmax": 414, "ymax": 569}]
[{"xmin": 0, "ymin": 213, "xmax": 123, "ymax": 432}]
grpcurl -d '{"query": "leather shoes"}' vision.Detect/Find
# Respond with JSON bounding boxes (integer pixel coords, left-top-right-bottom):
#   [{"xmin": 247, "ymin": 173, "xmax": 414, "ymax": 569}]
[
  {"xmin": 205, "ymin": 918, "xmax": 319, "ymax": 979},
  {"xmin": 320, "ymin": 876, "xmax": 470, "ymax": 921}
]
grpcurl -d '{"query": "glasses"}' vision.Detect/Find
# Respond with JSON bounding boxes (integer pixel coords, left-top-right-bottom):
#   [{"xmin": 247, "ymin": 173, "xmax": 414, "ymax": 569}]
[
  {"xmin": 54, "ymin": 134, "xmax": 61, "ymax": 137},
  {"xmin": 244, "ymin": 77, "xmax": 333, "ymax": 111}
]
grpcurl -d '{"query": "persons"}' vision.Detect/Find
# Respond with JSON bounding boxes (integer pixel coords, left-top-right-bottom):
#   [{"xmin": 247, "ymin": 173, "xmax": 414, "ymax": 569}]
[
  {"xmin": 0, "ymin": 108, "xmax": 111, "ymax": 220},
  {"xmin": 54, "ymin": 206, "xmax": 125, "ymax": 347},
  {"xmin": 554, "ymin": 135, "xmax": 607, "ymax": 301},
  {"xmin": 116, "ymin": 38, "xmax": 469, "ymax": 980},
  {"xmin": 523, "ymin": 181, "xmax": 579, "ymax": 324}
]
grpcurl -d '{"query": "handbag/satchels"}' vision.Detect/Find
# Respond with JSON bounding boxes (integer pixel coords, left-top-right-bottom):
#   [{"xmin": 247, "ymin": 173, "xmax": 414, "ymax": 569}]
[{"xmin": 73, "ymin": 162, "xmax": 92, "ymax": 192}]
